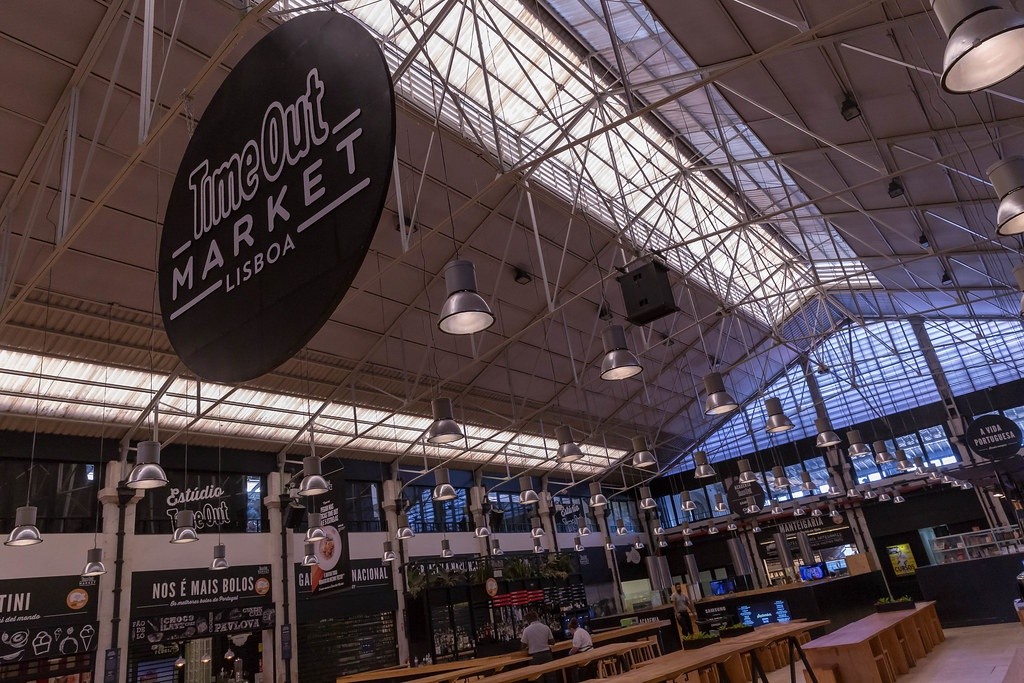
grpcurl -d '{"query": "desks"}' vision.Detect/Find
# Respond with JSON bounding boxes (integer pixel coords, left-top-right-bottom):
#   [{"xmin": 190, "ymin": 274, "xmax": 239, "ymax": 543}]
[
  {"xmin": 579, "ymin": 619, "xmax": 763, "ymax": 683},
  {"xmin": 400, "ymin": 657, "xmax": 534, "ymax": 683},
  {"xmin": 712, "ymin": 619, "xmax": 831, "ymax": 683},
  {"xmin": 474, "ymin": 641, "xmax": 655, "ymax": 683},
  {"xmin": 794, "ymin": 600, "xmax": 947, "ymax": 683}
]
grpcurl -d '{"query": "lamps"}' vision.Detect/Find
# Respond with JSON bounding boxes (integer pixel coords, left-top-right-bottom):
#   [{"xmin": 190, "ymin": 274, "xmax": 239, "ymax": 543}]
[
  {"xmin": 928, "ymin": 0, "xmax": 1024, "ymax": 95},
  {"xmin": 200, "ymin": 639, "xmax": 211, "ymax": 663},
  {"xmin": 208, "ymin": 407, "xmax": 229, "ymax": 570},
  {"xmin": 80, "ymin": 305, "xmax": 108, "ymax": 578},
  {"xmin": 1012, "ymin": 263, "xmax": 1024, "ymax": 320},
  {"xmin": 296, "ymin": 0, "xmax": 972, "ymax": 567},
  {"xmin": 986, "ymin": 155, "xmax": 1024, "ymax": 236},
  {"xmin": 224, "ymin": 635, "xmax": 234, "ymax": 659},
  {"xmin": 3, "ymin": 129, "xmax": 67, "ymax": 546},
  {"xmin": 169, "ymin": 368, "xmax": 199, "ymax": 545},
  {"xmin": 125, "ymin": 133, "xmax": 170, "ymax": 490},
  {"xmin": 174, "ymin": 653, "xmax": 186, "ymax": 667}
]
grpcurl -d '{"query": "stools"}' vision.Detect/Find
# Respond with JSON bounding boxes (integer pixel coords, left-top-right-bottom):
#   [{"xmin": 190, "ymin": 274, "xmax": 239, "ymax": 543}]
[{"xmin": 602, "ymin": 635, "xmax": 663, "ymax": 678}]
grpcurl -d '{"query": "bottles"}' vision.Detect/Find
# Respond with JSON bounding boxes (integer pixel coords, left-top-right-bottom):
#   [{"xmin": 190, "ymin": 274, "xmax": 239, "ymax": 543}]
[
  {"xmin": 477, "ymin": 613, "xmax": 560, "ymax": 641},
  {"xmin": 434, "ymin": 627, "xmax": 475, "ymax": 656},
  {"xmin": 414, "ymin": 656, "xmax": 419, "ymax": 667},
  {"xmin": 423, "ymin": 652, "xmax": 432, "ymax": 666}
]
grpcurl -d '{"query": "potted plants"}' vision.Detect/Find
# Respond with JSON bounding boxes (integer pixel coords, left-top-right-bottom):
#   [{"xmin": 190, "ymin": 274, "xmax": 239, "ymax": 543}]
[
  {"xmin": 716, "ymin": 622, "xmax": 754, "ymax": 639},
  {"xmin": 682, "ymin": 631, "xmax": 721, "ymax": 650},
  {"xmin": 874, "ymin": 595, "xmax": 916, "ymax": 614}
]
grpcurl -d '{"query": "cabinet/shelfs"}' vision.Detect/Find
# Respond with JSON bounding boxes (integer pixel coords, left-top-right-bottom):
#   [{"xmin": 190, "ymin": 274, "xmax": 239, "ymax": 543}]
[{"xmin": 927, "ymin": 523, "xmax": 1024, "ymax": 565}]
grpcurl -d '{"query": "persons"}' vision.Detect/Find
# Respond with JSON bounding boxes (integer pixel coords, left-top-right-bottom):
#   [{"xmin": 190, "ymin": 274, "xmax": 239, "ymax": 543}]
[
  {"xmin": 519, "ymin": 611, "xmax": 556, "ymax": 683},
  {"xmin": 568, "ymin": 618, "xmax": 597, "ymax": 682},
  {"xmin": 670, "ymin": 582, "xmax": 696, "ymax": 636}
]
